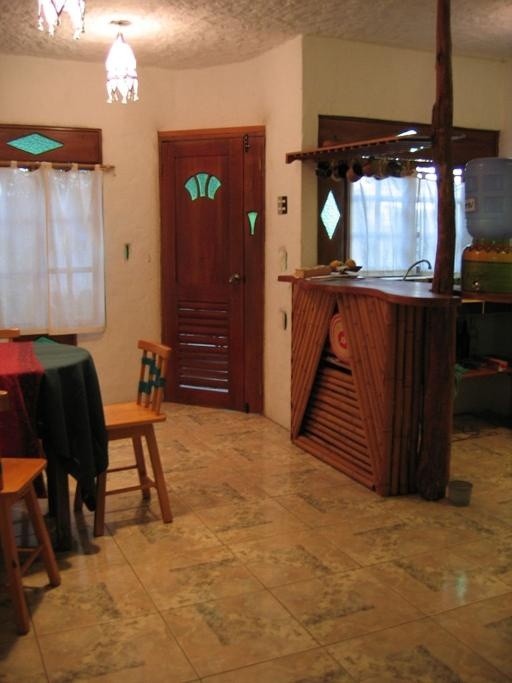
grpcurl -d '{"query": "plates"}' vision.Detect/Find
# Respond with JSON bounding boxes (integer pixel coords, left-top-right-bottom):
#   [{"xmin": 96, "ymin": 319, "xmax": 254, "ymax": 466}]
[{"xmin": 332, "ymin": 267, "xmax": 363, "ymax": 273}]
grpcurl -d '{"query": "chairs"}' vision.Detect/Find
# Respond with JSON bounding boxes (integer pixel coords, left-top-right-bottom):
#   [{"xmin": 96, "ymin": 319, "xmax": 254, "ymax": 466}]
[
  {"xmin": 1, "ymin": 391, "xmax": 62, "ymax": 637},
  {"xmin": 73, "ymin": 338, "xmax": 173, "ymax": 537}
]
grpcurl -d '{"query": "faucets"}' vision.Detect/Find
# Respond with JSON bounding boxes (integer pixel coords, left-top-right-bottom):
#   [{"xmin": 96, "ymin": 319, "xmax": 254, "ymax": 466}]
[{"xmin": 401, "ymin": 259, "xmax": 431, "ymax": 280}]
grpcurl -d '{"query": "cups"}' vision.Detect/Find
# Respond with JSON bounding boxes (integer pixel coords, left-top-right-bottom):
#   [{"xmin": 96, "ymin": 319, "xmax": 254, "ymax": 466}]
[{"xmin": 316, "ymin": 155, "xmax": 417, "ymax": 182}]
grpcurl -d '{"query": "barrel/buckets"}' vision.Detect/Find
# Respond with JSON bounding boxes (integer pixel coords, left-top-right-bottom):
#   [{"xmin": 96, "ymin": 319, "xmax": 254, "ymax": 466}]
[{"xmin": 463, "ymin": 159, "xmax": 512, "ymax": 245}]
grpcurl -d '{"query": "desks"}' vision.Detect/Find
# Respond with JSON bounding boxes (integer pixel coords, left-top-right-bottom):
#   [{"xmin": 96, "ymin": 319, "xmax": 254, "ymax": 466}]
[{"xmin": 0, "ymin": 340, "xmax": 90, "ymax": 551}]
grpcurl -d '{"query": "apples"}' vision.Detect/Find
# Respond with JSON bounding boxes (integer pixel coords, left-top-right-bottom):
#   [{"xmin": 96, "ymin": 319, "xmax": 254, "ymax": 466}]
[
  {"xmin": 330, "ymin": 260, "xmax": 343, "ymax": 272},
  {"xmin": 344, "ymin": 259, "xmax": 356, "ymax": 268}
]
